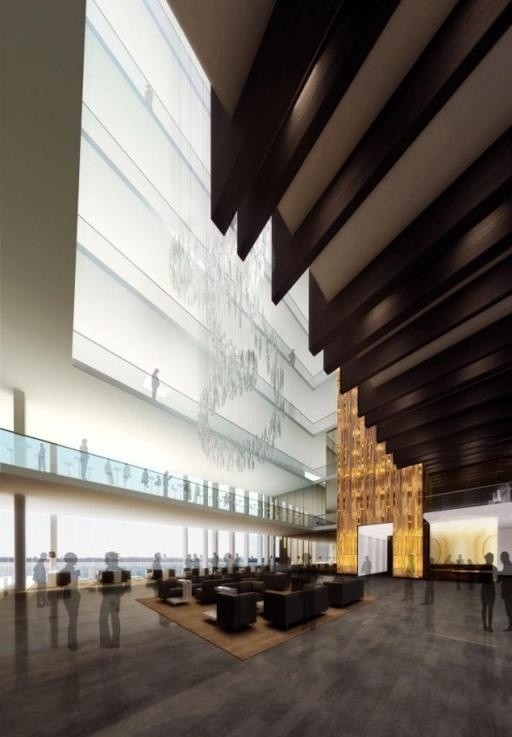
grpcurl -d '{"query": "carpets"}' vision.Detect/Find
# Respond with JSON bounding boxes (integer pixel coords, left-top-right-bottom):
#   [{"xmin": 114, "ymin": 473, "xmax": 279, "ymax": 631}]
[{"xmin": 134, "ymin": 581, "xmax": 374, "ymax": 662}]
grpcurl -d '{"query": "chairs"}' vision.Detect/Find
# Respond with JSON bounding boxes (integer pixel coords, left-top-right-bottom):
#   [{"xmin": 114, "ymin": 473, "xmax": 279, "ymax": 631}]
[{"xmin": 55, "ymin": 562, "xmax": 338, "ymax": 634}]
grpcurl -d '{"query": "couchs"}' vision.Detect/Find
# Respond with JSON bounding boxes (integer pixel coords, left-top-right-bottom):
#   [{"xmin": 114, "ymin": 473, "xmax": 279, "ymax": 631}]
[{"xmin": 323, "ymin": 579, "xmax": 365, "ymax": 608}]
